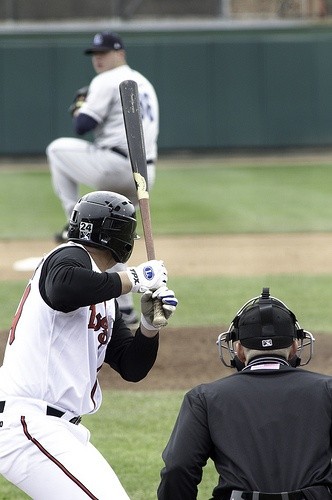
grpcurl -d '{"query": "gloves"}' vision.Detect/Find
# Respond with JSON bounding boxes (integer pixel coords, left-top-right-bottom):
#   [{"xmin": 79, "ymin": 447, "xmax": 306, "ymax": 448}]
[
  {"xmin": 139, "ymin": 286, "xmax": 178, "ymax": 331},
  {"xmin": 127, "ymin": 261, "xmax": 167, "ymax": 292}
]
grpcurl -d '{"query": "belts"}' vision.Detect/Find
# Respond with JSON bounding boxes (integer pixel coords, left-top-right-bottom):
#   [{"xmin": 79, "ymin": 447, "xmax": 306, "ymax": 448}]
[
  {"xmin": 212, "ymin": 488, "xmax": 307, "ymax": 500},
  {"xmin": 0, "ymin": 399, "xmax": 82, "ymax": 425},
  {"xmin": 103, "ymin": 146, "xmax": 153, "ymax": 165}
]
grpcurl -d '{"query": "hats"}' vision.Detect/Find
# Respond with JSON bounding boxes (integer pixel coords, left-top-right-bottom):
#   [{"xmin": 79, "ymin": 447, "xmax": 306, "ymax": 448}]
[
  {"xmin": 238, "ymin": 305, "xmax": 294, "ymax": 350},
  {"xmin": 83, "ymin": 32, "xmax": 123, "ymax": 57}
]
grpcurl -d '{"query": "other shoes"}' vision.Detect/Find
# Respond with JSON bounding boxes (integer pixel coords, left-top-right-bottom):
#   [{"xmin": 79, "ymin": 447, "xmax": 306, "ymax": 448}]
[{"xmin": 55, "ymin": 225, "xmax": 68, "ymax": 242}]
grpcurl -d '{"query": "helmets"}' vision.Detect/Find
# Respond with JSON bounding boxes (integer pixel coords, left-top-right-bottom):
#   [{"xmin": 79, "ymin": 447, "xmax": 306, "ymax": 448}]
[{"xmin": 68, "ymin": 191, "xmax": 140, "ymax": 264}]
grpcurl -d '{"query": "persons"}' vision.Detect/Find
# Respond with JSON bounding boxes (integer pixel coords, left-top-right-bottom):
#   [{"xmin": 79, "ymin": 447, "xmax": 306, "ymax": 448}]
[
  {"xmin": 0, "ymin": 190, "xmax": 178, "ymax": 500},
  {"xmin": 45, "ymin": 31, "xmax": 159, "ymax": 323},
  {"xmin": 157, "ymin": 287, "xmax": 332, "ymax": 500}
]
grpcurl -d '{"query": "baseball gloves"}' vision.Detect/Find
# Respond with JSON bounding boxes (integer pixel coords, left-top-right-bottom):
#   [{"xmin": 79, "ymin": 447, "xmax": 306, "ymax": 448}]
[{"xmin": 68, "ymin": 85, "xmax": 89, "ymax": 116}]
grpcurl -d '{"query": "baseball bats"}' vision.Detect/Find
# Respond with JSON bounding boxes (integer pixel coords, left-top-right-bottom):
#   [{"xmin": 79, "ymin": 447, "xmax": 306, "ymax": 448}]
[{"xmin": 118, "ymin": 81, "xmax": 169, "ymax": 329}]
